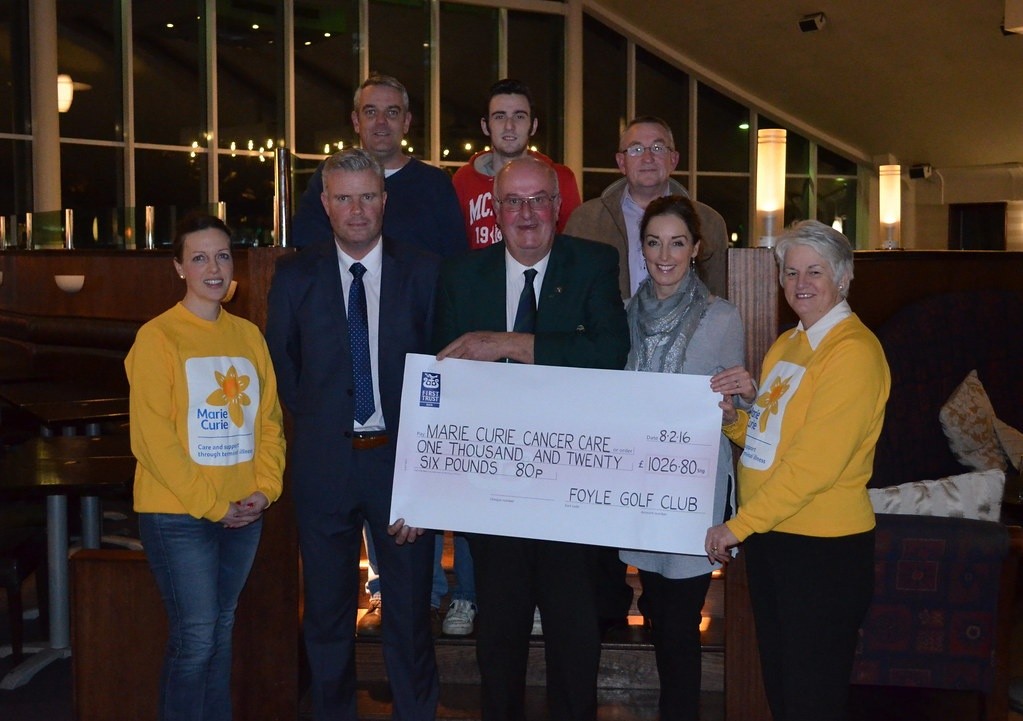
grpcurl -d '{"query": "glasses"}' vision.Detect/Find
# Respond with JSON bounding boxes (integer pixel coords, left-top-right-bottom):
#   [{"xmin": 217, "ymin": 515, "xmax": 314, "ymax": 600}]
[
  {"xmin": 623, "ymin": 143, "xmax": 674, "ymax": 156},
  {"xmin": 495, "ymin": 194, "xmax": 556, "ymax": 212}
]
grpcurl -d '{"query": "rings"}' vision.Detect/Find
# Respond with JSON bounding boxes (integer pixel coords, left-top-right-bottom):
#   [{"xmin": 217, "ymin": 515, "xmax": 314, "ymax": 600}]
[
  {"xmin": 712, "ymin": 547, "xmax": 717, "ymax": 551},
  {"xmin": 735, "ymin": 381, "xmax": 739, "ymax": 388}
]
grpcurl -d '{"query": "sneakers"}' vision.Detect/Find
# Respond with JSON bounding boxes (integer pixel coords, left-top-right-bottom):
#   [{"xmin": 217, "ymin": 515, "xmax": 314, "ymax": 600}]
[
  {"xmin": 532, "ymin": 607, "xmax": 543, "ymax": 636},
  {"xmin": 443, "ymin": 599, "xmax": 478, "ymax": 636}
]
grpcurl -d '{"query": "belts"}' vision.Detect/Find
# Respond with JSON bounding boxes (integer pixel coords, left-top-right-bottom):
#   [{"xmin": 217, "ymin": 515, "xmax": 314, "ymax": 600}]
[{"xmin": 353, "ymin": 435, "xmax": 389, "ymax": 450}]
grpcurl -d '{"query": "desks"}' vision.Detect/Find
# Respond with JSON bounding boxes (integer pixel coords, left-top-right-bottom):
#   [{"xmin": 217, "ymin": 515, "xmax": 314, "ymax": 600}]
[
  {"xmin": 0, "ymin": 430, "xmax": 136, "ymax": 692},
  {"xmin": 0, "ymin": 374, "xmax": 129, "ymax": 560}
]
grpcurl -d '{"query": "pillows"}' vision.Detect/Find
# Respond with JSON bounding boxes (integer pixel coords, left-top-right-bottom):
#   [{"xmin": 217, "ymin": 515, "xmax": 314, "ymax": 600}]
[
  {"xmin": 868, "ymin": 467, "xmax": 1006, "ymax": 522},
  {"xmin": 936, "ymin": 368, "xmax": 1010, "ymax": 471},
  {"xmin": 992, "ymin": 416, "xmax": 1023, "ymax": 468}
]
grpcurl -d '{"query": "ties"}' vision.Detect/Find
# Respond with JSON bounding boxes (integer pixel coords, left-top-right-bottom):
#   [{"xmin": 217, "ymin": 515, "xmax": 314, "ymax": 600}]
[
  {"xmin": 348, "ymin": 262, "xmax": 375, "ymax": 425},
  {"xmin": 508, "ymin": 269, "xmax": 537, "ymax": 363}
]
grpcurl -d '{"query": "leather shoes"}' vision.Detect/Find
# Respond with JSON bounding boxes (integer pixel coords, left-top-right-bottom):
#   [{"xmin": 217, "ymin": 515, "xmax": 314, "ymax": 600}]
[
  {"xmin": 357, "ymin": 599, "xmax": 383, "ymax": 635},
  {"xmin": 431, "ymin": 606, "xmax": 440, "ymax": 638}
]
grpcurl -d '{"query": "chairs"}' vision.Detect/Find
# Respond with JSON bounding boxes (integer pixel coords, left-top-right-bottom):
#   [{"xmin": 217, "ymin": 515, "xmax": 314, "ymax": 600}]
[{"xmin": 848, "ymin": 513, "xmax": 1023, "ymax": 721}]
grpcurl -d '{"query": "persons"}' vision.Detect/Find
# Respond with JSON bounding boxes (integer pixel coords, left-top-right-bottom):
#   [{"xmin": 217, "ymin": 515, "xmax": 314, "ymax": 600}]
[
  {"xmin": 450, "ymin": 77, "xmax": 580, "ymax": 639},
  {"xmin": 294, "ymin": 75, "xmax": 465, "ymax": 640},
  {"xmin": 619, "ymin": 194, "xmax": 757, "ymax": 721},
  {"xmin": 564, "ymin": 117, "xmax": 729, "ymax": 635},
  {"xmin": 266, "ymin": 151, "xmax": 447, "ymax": 721},
  {"xmin": 387, "ymin": 158, "xmax": 632, "ymax": 721},
  {"xmin": 706, "ymin": 220, "xmax": 891, "ymax": 721},
  {"xmin": 124, "ymin": 215, "xmax": 287, "ymax": 721}
]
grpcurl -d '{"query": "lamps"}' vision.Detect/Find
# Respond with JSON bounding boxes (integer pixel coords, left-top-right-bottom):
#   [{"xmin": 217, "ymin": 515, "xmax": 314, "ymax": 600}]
[
  {"xmin": 753, "ymin": 128, "xmax": 788, "ymax": 249},
  {"xmin": 874, "ymin": 164, "xmax": 905, "ymax": 250}
]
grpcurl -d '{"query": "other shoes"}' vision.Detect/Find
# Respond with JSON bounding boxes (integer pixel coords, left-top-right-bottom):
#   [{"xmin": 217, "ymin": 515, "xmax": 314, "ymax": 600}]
[{"xmin": 602, "ymin": 618, "xmax": 628, "ymax": 643}]
